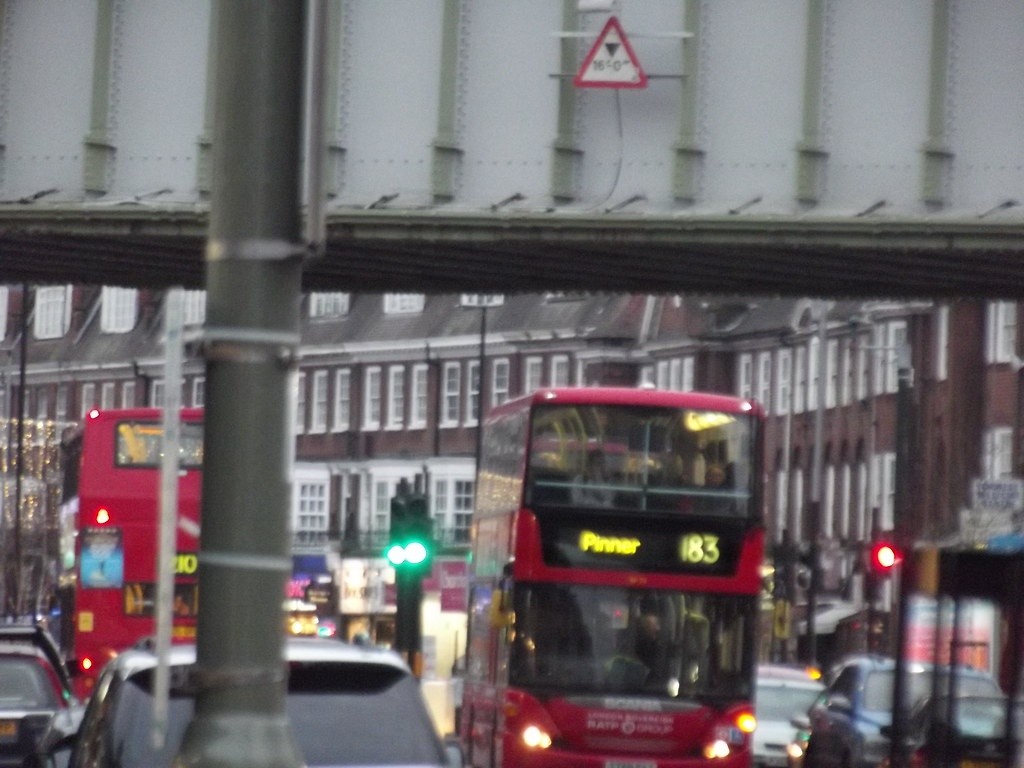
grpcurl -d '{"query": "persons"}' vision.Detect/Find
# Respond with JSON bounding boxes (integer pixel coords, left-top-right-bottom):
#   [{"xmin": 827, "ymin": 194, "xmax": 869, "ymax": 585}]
[
  {"xmin": 697, "ymin": 467, "xmax": 732, "ymax": 513},
  {"xmin": 631, "ymin": 613, "xmax": 668, "ymax": 666},
  {"xmin": 570, "ymin": 450, "xmax": 620, "ymax": 507}
]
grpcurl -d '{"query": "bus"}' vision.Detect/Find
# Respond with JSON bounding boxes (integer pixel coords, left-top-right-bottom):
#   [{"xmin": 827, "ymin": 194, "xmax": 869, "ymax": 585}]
[
  {"xmin": 54, "ymin": 405, "xmax": 203, "ymax": 701},
  {"xmin": 456, "ymin": 384, "xmax": 767, "ymax": 768}
]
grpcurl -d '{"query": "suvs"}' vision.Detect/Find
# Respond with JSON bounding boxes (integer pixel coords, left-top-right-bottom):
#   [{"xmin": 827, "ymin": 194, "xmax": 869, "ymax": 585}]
[
  {"xmin": 786, "ymin": 651, "xmax": 1006, "ymax": 768},
  {"xmin": 1, "ymin": 624, "xmax": 70, "ymax": 691},
  {"xmin": 66, "ymin": 634, "xmax": 451, "ymax": 767}
]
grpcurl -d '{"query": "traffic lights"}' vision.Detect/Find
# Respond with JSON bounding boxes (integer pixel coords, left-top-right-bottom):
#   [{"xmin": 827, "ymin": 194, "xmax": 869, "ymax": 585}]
[
  {"xmin": 381, "ymin": 493, "xmax": 407, "ymax": 580},
  {"xmin": 403, "ymin": 496, "xmax": 435, "ymax": 578},
  {"xmin": 862, "ymin": 538, "xmax": 898, "ymax": 608}
]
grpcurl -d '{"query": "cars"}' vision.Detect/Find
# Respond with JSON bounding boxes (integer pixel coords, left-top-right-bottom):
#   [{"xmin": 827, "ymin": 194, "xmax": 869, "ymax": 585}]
[
  {"xmin": 877, "ymin": 687, "xmax": 1023, "ymax": 768},
  {"xmin": 0, "ymin": 642, "xmax": 68, "ymax": 766},
  {"xmin": 753, "ymin": 662, "xmax": 826, "ymax": 767}
]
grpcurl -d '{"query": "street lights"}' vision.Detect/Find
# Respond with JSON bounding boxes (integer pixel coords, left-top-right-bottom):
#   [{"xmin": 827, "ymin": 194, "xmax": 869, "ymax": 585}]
[{"xmin": 457, "ymin": 290, "xmax": 505, "ymax": 496}]
